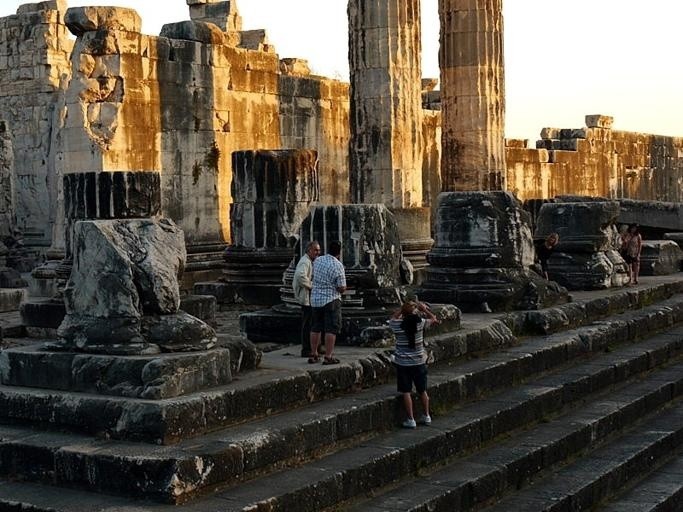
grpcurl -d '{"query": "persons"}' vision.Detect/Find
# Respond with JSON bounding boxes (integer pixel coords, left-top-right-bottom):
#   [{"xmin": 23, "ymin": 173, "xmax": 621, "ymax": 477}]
[
  {"xmin": 305, "ymin": 239, "xmax": 348, "ymax": 365},
  {"xmin": 530, "ymin": 232, "xmax": 559, "ymax": 281},
  {"xmin": 619, "ymin": 224, "xmax": 629, "ymax": 264},
  {"xmin": 291, "ymin": 239, "xmax": 327, "ymax": 358},
  {"xmin": 624, "ymin": 221, "xmax": 642, "ymax": 284},
  {"xmin": 388, "ymin": 297, "xmax": 439, "ymax": 429}
]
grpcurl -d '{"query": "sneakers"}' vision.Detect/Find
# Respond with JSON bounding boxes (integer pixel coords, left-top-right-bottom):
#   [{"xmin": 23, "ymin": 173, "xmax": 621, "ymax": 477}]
[
  {"xmin": 300, "ymin": 349, "xmax": 335, "ymax": 357},
  {"xmin": 420, "ymin": 415, "xmax": 432, "ymax": 425},
  {"xmin": 404, "ymin": 419, "xmax": 417, "ymax": 429}
]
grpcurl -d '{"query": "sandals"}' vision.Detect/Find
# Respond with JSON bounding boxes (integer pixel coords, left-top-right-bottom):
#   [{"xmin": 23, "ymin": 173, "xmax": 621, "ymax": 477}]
[{"xmin": 307, "ymin": 354, "xmax": 340, "ymax": 364}]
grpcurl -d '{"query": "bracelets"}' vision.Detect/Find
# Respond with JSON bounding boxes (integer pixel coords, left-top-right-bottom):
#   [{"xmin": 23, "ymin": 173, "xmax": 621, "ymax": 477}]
[{"xmin": 423, "ymin": 308, "xmax": 426, "ymax": 313}]
